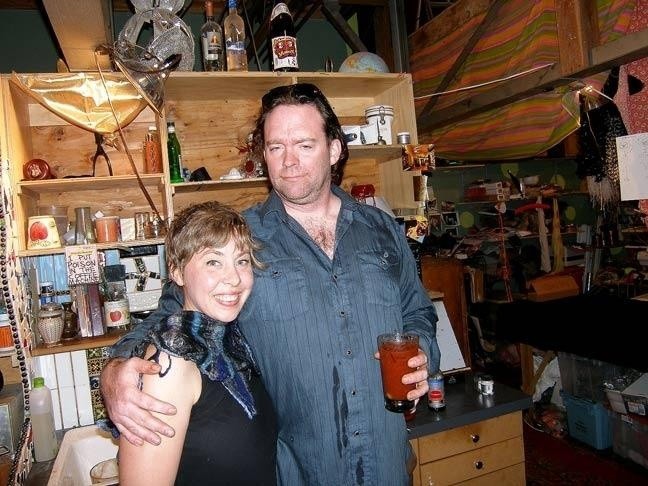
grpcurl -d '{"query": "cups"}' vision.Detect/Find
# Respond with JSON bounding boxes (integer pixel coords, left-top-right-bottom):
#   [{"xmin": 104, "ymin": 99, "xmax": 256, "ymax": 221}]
[
  {"xmin": 376, "ymin": 331, "xmax": 421, "ymax": 413},
  {"xmin": 104, "ymin": 300, "xmax": 131, "ymax": 326},
  {"xmin": 93, "ymin": 216, "xmax": 120, "ymax": 241},
  {"xmin": 90, "ymin": 457, "xmax": 120, "ymax": 484},
  {"xmin": 26, "ymin": 203, "xmax": 69, "ymax": 248}
]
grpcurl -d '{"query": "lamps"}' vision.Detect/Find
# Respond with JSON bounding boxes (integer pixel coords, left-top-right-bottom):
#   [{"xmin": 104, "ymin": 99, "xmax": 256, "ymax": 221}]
[{"xmin": 616, "ymin": 133, "xmax": 647, "ymax": 201}]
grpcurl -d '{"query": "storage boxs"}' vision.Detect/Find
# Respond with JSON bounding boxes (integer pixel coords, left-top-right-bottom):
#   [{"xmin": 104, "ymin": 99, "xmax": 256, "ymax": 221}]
[
  {"xmin": 563, "ymin": 393, "xmax": 614, "ymax": 450},
  {"xmin": 602, "ymin": 367, "xmax": 646, "ymax": 466}
]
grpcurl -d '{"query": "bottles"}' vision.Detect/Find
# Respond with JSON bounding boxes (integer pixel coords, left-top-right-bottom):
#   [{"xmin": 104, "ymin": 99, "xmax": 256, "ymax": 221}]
[
  {"xmin": 268, "ymin": 0, "xmax": 300, "ymax": 72},
  {"xmin": 473, "ymin": 375, "xmax": 494, "ymax": 398},
  {"xmin": 135, "ymin": 211, "xmax": 161, "ymax": 238},
  {"xmin": 38, "ymin": 282, "xmax": 81, "ymax": 347},
  {"xmin": 74, "ymin": 206, "xmax": 96, "ymax": 245},
  {"xmin": 200, "ymin": 0, "xmax": 222, "ymax": 71},
  {"xmin": 396, "ymin": 131, "xmax": 409, "ymax": 145},
  {"xmin": 343, "ymin": 133, "xmax": 357, "ymax": 143},
  {"xmin": 224, "ymin": 0, "xmax": 249, "ymax": 70},
  {"xmin": 145, "ymin": 126, "xmax": 161, "ymax": 173},
  {"xmin": 167, "ymin": 121, "xmax": 184, "ymax": 183}
]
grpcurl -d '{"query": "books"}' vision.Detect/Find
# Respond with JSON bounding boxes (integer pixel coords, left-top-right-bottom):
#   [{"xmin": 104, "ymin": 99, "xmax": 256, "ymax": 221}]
[{"xmin": 454, "ymin": 239, "xmax": 482, "ymax": 259}]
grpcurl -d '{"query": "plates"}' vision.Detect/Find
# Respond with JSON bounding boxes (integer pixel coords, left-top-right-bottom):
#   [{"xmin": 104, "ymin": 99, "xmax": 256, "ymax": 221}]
[{"xmin": 23, "ymin": 158, "xmax": 50, "ymax": 180}]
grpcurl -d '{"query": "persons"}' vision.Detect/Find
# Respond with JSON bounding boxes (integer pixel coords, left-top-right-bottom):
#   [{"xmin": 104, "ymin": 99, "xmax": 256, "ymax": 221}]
[
  {"xmin": 119, "ymin": 201, "xmax": 281, "ymax": 486},
  {"xmin": 100, "ymin": 84, "xmax": 441, "ymax": 485}
]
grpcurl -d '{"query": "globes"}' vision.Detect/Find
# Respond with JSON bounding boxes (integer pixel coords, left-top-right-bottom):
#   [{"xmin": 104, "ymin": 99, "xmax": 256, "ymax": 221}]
[{"xmin": 337, "ymin": 51, "xmax": 391, "ymax": 72}]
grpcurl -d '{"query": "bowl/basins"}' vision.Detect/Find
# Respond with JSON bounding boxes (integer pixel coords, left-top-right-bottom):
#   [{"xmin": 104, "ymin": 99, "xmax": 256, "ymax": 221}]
[
  {"xmin": 518, "ymin": 174, "xmax": 541, "ymax": 186},
  {"xmin": 351, "ymin": 184, "xmax": 376, "ymax": 197}
]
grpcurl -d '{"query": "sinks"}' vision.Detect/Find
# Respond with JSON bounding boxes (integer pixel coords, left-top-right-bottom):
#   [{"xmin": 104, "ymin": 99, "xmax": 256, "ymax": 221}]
[{"xmin": 45, "ymin": 424, "xmax": 120, "ymax": 486}]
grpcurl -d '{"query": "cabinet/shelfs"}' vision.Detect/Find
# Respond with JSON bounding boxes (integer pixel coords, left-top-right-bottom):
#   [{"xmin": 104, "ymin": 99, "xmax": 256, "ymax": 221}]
[
  {"xmin": 150, "ymin": 72, "xmax": 425, "ymax": 229},
  {"xmin": 417, "ymin": 411, "xmax": 528, "ymax": 485},
  {"xmin": 1, "ymin": 71, "xmax": 173, "ymax": 361}
]
